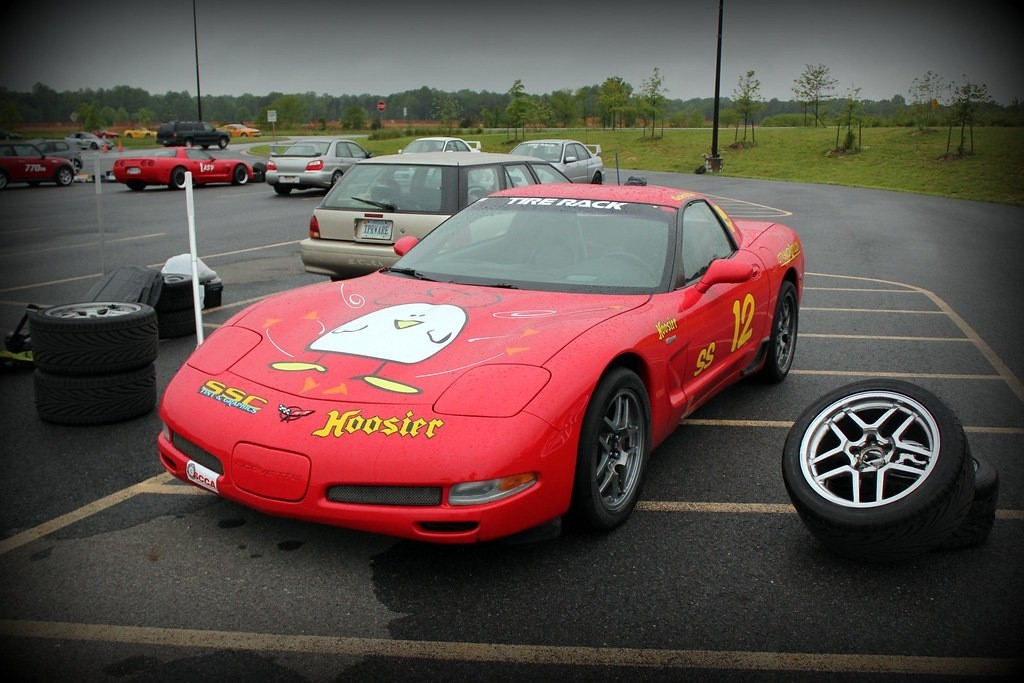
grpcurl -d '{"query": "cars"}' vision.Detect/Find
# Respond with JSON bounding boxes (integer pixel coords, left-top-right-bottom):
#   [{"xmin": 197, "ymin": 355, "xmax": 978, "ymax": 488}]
[
  {"xmin": 0, "ymin": 129, "xmax": 22, "ymax": 143},
  {"xmin": 0, "ymin": 141, "xmax": 78, "ymax": 190},
  {"xmin": 390, "ymin": 136, "xmax": 483, "ymax": 187},
  {"xmin": 500, "ymin": 138, "xmax": 607, "ymax": 199},
  {"xmin": 65, "ymin": 132, "xmax": 114, "ymax": 150},
  {"xmin": 216, "ymin": 124, "xmax": 261, "ymax": 138},
  {"xmin": 264, "ymin": 136, "xmax": 380, "ymax": 194},
  {"xmin": 94, "ymin": 130, "xmax": 119, "ymax": 139},
  {"xmin": 298, "ymin": 150, "xmax": 575, "ymax": 287},
  {"xmin": 123, "ymin": 127, "xmax": 157, "ymax": 138},
  {"xmin": 22, "ymin": 139, "xmax": 84, "ymax": 176}
]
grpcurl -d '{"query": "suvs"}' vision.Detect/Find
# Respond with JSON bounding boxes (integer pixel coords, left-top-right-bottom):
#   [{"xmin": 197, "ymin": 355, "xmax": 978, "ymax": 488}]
[{"xmin": 156, "ymin": 121, "xmax": 230, "ymax": 149}]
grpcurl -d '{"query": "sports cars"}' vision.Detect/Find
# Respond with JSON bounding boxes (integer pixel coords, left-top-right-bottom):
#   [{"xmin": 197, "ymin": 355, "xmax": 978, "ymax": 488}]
[
  {"xmin": 112, "ymin": 146, "xmax": 255, "ymax": 189},
  {"xmin": 153, "ymin": 182, "xmax": 808, "ymax": 548}
]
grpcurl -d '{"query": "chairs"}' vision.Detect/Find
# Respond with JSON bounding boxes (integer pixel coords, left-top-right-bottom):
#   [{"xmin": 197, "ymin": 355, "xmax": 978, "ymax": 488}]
[
  {"xmin": 370, "ymin": 184, "xmax": 394, "ymax": 200},
  {"xmin": 487, "ymin": 212, "xmax": 585, "ymax": 272}
]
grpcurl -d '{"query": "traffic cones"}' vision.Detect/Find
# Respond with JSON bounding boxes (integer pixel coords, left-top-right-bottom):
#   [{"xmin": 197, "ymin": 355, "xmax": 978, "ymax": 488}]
[
  {"xmin": 102, "ymin": 141, "xmax": 108, "ymax": 153},
  {"xmin": 117, "ymin": 140, "xmax": 123, "ymax": 152}
]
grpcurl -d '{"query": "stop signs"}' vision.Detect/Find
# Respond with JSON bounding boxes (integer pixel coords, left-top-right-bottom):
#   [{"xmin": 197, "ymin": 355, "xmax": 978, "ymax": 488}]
[{"xmin": 377, "ymin": 101, "xmax": 385, "ymax": 112}]
